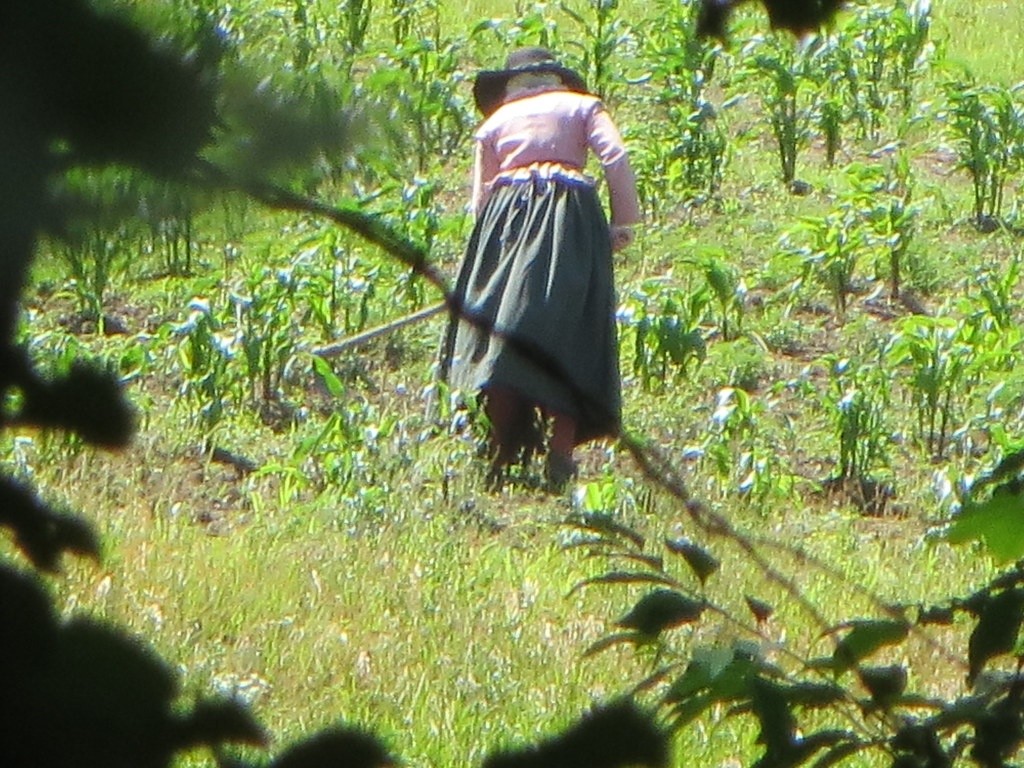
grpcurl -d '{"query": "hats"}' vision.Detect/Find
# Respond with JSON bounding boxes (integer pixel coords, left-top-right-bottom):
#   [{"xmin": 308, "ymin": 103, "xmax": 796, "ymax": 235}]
[{"xmin": 473, "ymin": 49, "xmax": 587, "ymax": 116}]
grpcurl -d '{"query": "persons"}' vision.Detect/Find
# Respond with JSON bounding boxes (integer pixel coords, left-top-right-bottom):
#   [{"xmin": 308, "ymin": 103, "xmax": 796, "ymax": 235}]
[{"xmin": 430, "ymin": 49, "xmax": 639, "ymax": 506}]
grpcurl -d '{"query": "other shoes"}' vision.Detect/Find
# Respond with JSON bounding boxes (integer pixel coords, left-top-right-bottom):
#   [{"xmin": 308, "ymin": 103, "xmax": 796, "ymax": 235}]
[{"xmin": 543, "ymin": 453, "xmax": 578, "ymax": 484}]
